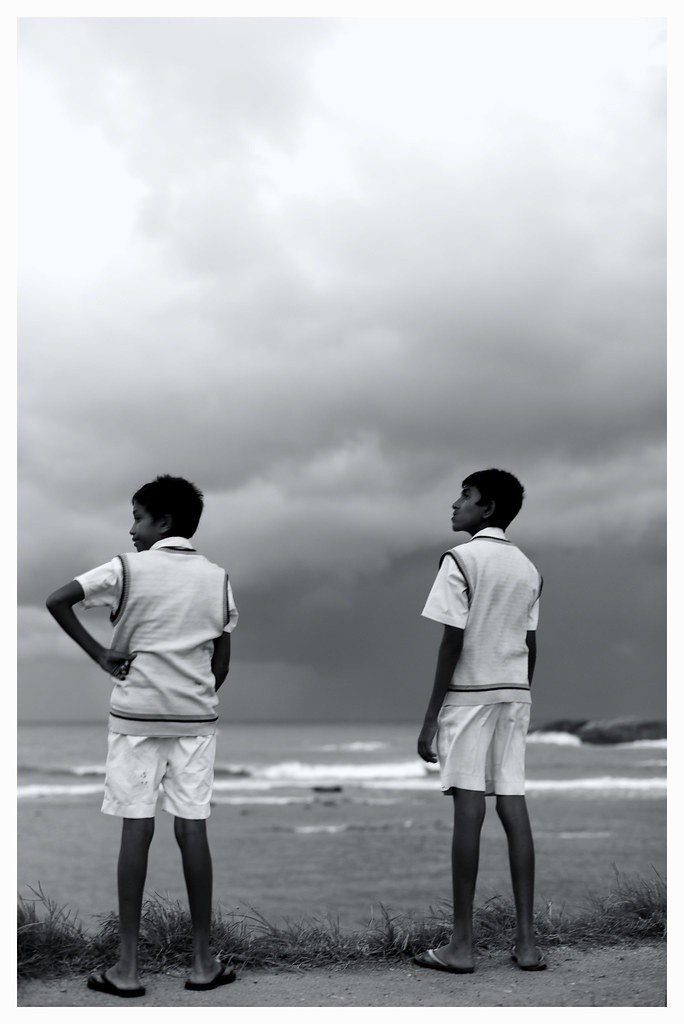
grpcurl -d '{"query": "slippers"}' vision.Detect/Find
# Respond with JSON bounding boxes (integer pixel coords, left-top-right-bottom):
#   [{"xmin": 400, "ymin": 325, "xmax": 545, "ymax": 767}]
[
  {"xmin": 414, "ymin": 949, "xmax": 475, "ymax": 974},
  {"xmin": 185, "ymin": 964, "xmax": 236, "ymax": 990},
  {"xmin": 512, "ymin": 946, "xmax": 547, "ymax": 971},
  {"xmin": 88, "ymin": 970, "xmax": 146, "ymax": 997}
]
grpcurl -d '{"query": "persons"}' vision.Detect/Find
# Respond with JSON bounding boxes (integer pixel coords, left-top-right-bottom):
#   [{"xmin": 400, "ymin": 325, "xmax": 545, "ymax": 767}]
[
  {"xmin": 414, "ymin": 468, "xmax": 546, "ymax": 974},
  {"xmin": 46, "ymin": 475, "xmax": 238, "ymax": 998}
]
